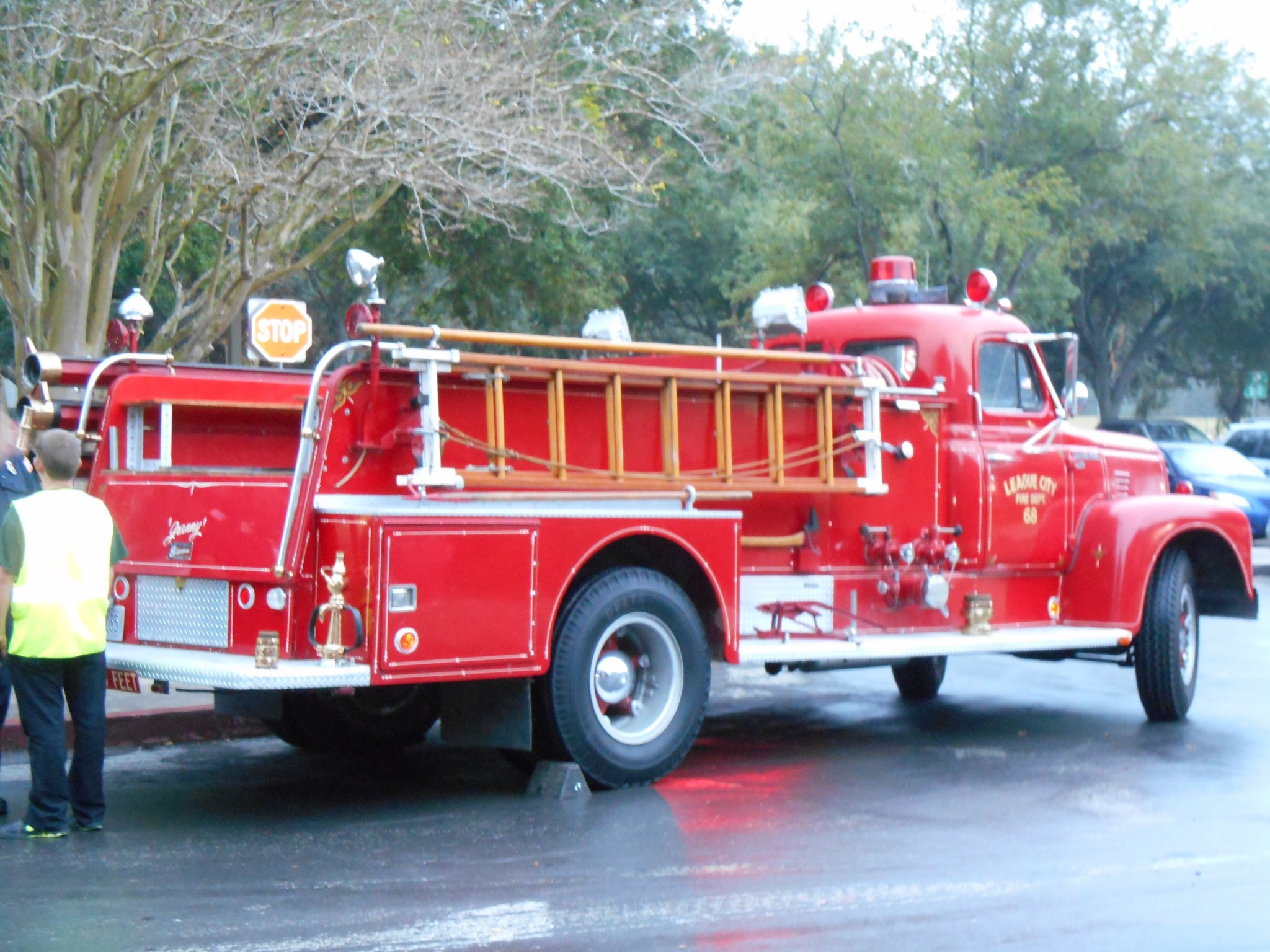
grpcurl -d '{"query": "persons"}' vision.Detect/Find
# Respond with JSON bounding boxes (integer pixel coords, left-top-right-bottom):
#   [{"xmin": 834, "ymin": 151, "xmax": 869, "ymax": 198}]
[
  {"xmin": 0, "ymin": 412, "xmax": 42, "ymax": 815},
  {"xmin": 1, "ymin": 429, "xmax": 128, "ymax": 839}
]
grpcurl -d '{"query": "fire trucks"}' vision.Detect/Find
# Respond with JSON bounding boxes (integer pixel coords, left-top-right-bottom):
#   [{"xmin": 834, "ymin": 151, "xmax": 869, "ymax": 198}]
[{"xmin": 69, "ymin": 255, "xmax": 1257, "ymax": 787}]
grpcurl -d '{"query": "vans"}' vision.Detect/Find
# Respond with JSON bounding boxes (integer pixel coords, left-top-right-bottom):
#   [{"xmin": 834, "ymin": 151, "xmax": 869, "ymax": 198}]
[{"xmin": 1097, "ymin": 420, "xmax": 1210, "ymax": 447}]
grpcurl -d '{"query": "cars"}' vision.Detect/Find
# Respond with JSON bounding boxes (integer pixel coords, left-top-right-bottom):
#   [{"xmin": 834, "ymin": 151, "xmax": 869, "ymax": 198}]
[
  {"xmin": 1215, "ymin": 422, "xmax": 1270, "ymax": 482},
  {"xmin": 1158, "ymin": 441, "xmax": 1269, "ymax": 539}
]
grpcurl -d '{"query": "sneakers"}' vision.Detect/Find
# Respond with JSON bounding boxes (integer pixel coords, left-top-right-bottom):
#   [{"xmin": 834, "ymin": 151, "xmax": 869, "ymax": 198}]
[
  {"xmin": 69, "ymin": 816, "xmax": 103, "ymax": 831},
  {"xmin": 0, "ymin": 818, "xmax": 68, "ymax": 839}
]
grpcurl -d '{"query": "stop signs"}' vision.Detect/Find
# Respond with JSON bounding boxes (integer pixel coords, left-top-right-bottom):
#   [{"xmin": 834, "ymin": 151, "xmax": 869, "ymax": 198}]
[{"xmin": 250, "ymin": 300, "xmax": 313, "ymax": 364}]
